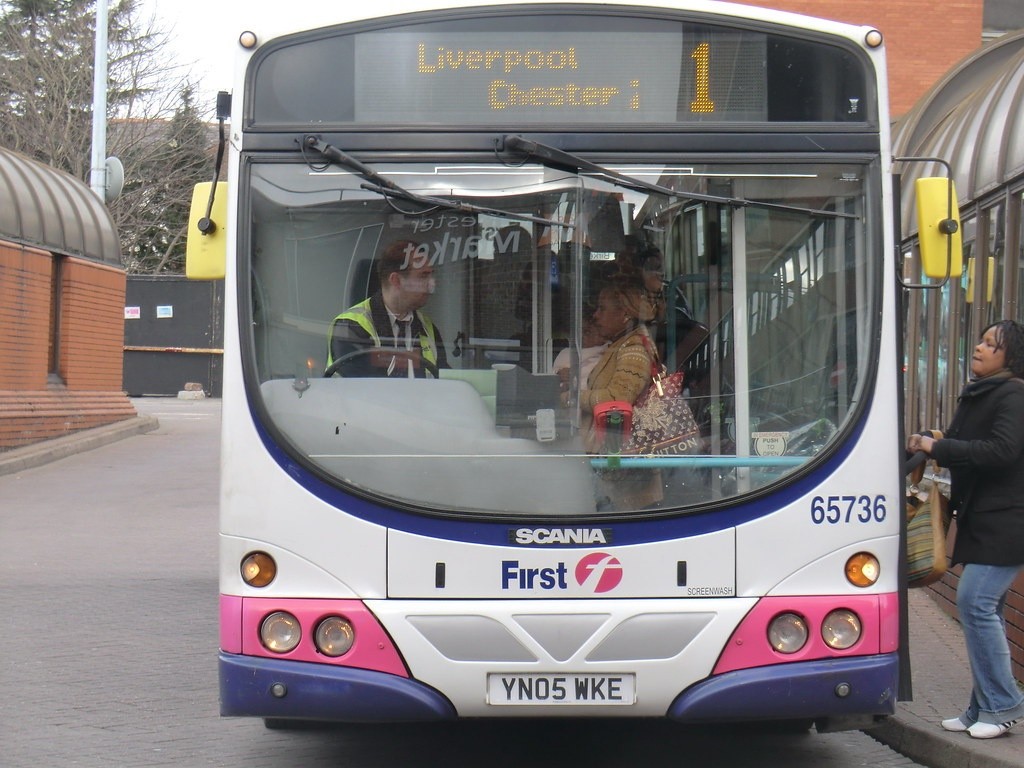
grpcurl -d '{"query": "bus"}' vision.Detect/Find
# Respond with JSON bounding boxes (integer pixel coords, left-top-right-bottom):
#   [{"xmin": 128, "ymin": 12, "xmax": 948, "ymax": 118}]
[{"xmin": 185, "ymin": 0, "xmax": 966, "ymax": 731}]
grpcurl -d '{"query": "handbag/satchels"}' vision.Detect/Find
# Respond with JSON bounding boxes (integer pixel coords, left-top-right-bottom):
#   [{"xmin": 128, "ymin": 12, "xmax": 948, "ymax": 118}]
[
  {"xmin": 621, "ymin": 334, "xmax": 706, "ymax": 455},
  {"xmin": 905, "ymin": 430, "xmax": 954, "ymax": 587}
]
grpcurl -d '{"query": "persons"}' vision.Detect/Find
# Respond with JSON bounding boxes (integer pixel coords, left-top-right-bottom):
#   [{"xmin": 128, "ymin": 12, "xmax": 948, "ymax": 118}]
[
  {"xmin": 325, "ymin": 238, "xmax": 451, "ymax": 378},
  {"xmin": 518, "ymin": 238, "xmax": 692, "ymax": 511},
  {"xmin": 907, "ymin": 320, "xmax": 1024, "ymax": 739}
]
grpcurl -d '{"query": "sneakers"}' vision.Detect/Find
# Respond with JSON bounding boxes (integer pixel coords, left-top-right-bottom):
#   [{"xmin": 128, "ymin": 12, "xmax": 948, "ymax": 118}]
[
  {"xmin": 966, "ymin": 712, "xmax": 1024, "ymax": 738},
  {"xmin": 941, "ymin": 718, "xmax": 967, "ymax": 732}
]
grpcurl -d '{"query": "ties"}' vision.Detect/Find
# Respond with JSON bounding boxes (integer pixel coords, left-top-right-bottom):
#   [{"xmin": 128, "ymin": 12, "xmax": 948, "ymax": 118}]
[{"xmin": 394, "ymin": 318, "xmax": 408, "ymax": 377}]
